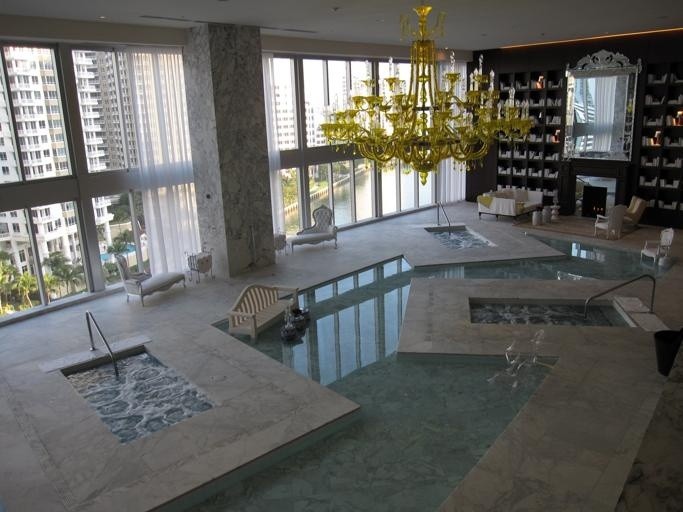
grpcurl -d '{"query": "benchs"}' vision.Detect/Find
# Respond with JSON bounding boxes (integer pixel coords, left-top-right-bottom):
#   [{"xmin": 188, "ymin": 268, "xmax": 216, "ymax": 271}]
[{"xmin": 227, "ymin": 285, "xmax": 301, "ymax": 346}]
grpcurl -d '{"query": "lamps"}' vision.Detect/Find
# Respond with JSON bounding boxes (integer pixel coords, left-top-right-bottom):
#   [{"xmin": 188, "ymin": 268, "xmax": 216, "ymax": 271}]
[{"xmin": 321, "ymin": 1, "xmax": 537, "ymax": 184}]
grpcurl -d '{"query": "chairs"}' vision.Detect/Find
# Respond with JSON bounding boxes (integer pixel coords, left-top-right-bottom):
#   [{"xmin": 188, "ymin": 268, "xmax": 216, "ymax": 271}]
[
  {"xmin": 115, "ymin": 254, "xmax": 187, "ymax": 307},
  {"xmin": 621, "ymin": 196, "xmax": 647, "ymax": 233},
  {"xmin": 594, "ymin": 204, "xmax": 627, "ymax": 241},
  {"xmin": 640, "ymin": 228, "xmax": 674, "ymax": 264},
  {"xmin": 286, "ymin": 204, "xmax": 337, "ymax": 254}
]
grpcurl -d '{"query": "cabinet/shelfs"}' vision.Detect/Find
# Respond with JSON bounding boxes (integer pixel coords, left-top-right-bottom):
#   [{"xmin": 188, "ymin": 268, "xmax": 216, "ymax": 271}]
[
  {"xmin": 630, "ymin": 64, "xmax": 683, "ymax": 230},
  {"xmin": 493, "ymin": 66, "xmax": 565, "ymax": 216}
]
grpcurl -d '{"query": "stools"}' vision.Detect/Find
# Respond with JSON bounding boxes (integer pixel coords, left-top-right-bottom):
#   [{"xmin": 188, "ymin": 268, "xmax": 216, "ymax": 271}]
[
  {"xmin": 532, "ymin": 210, "xmax": 543, "ymax": 226},
  {"xmin": 542, "ymin": 206, "xmax": 552, "ymax": 223}
]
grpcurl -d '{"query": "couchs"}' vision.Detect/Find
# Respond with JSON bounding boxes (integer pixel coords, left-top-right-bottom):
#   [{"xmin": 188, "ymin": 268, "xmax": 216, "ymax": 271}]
[{"xmin": 477, "ymin": 190, "xmax": 543, "ymax": 226}]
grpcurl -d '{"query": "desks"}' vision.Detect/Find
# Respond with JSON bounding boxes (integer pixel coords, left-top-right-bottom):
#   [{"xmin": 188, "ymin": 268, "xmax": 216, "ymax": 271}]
[
  {"xmin": 188, "ymin": 248, "xmax": 215, "ymax": 283},
  {"xmin": 274, "ymin": 234, "xmax": 288, "ymax": 256}
]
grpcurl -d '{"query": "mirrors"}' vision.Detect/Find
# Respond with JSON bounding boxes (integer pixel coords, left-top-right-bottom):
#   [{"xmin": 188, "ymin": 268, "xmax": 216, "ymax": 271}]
[{"xmin": 562, "ymin": 51, "xmax": 642, "ymax": 162}]
